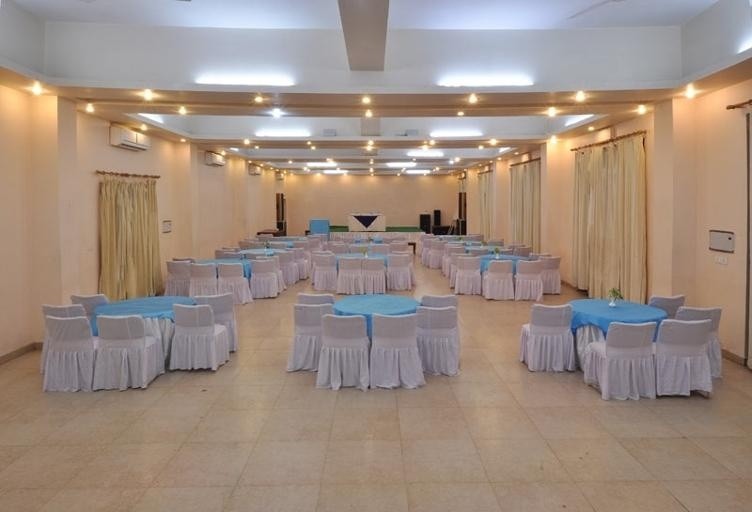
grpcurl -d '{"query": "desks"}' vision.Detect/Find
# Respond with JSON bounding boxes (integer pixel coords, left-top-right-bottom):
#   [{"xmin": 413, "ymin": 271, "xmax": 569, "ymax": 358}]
[{"xmin": 348, "ymin": 214, "xmax": 386, "ymax": 232}]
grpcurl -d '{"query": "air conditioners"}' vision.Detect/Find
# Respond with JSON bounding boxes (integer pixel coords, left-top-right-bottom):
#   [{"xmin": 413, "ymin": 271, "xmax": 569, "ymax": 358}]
[
  {"xmin": 249, "ymin": 164, "xmax": 261, "ymax": 175},
  {"xmin": 110, "ymin": 126, "xmax": 148, "ymax": 151},
  {"xmin": 277, "ymin": 173, "xmax": 284, "ymax": 180},
  {"xmin": 205, "ymin": 151, "xmax": 225, "ymax": 166}
]
